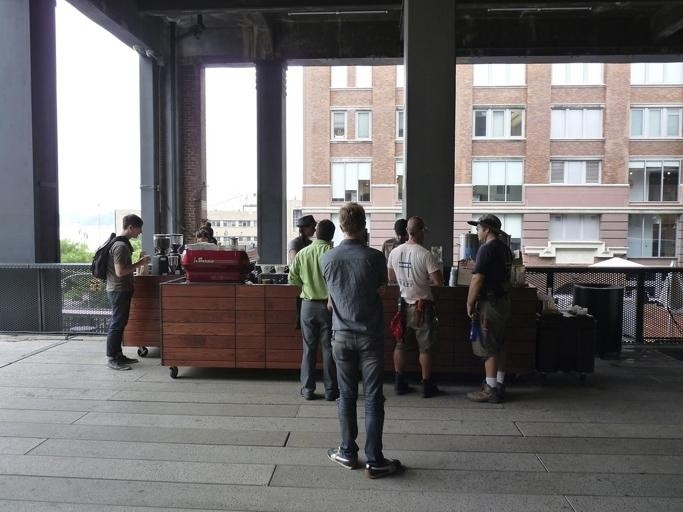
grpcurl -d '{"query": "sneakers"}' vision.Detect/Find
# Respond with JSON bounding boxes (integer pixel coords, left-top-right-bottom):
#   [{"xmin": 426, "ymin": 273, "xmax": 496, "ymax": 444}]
[
  {"xmin": 117, "ymin": 355, "xmax": 138, "ymax": 364},
  {"xmin": 301, "ymin": 385, "xmax": 313, "ymax": 400},
  {"xmin": 422, "ymin": 386, "xmax": 447, "ymax": 398},
  {"xmin": 326, "ymin": 389, "xmax": 340, "ymax": 401},
  {"xmin": 365, "ymin": 458, "xmax": 401, "ymax": 479},
  {"xmin": 465, "ymin": 380, "xmax": 506, "ymax": 403},
  {"xmin": 394, "ymin": 383, "xmax": 416, "ymax": 395},
  {"xmin": 327, "ymin": 446, "xmax": 356, "ymax": 470},
  {"xmin": 108, "ymin": 359, "xmax": 130, "ymax": 371}
]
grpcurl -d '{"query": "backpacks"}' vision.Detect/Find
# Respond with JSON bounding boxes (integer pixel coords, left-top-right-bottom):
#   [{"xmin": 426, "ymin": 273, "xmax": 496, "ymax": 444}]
[{"xmin": 90, "ymin": 233, "xmax": 134, "ymax": 279}]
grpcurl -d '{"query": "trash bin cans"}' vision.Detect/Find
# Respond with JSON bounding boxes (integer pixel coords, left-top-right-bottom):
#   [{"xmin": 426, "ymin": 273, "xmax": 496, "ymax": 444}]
[{"xmin": 571, "ymin": 284, "xmax": 624, "ymax": 360}]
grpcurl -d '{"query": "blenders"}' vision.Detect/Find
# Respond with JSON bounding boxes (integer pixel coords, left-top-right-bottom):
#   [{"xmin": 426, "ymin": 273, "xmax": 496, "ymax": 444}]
[
  {"xmin": 169, "ymin": 234, "xmax": 185, "ymax": 275},
  {"xmin": 150, "ymin": 234, "xmax": 171, "ymax": 277}
]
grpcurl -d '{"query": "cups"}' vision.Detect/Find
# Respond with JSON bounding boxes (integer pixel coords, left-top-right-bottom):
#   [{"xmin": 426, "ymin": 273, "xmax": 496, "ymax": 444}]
[
  {"xmin": 567, "ymin": 303, "xmax": 588, "ymax": 316},
  {"xmin": 230, "ymin": 237, "xmax": 239, "ymax": 250},
  {"xmin": 134, "ymin": 250, "xmax": 151, "ymax": 277},
  {"xmin": 261, "ymin": 265, "xmax": 273, "ymax": 274},
  {"xmin": 274, "ymin": 266, "xmax": 288, "ymax": 274},
  {"xmin": 261, "ymin": 279, "xmax": 273, "ymax": 284}
]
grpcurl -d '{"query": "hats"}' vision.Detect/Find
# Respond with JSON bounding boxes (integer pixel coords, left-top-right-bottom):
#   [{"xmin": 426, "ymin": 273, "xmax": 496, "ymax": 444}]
[
  {"xmin": 467, "ymin": 214, "xmax": 501, "ymax": 228},
  {"xmin": 395, "ymin": 219, "xmax": 408, "ymax": 235},
  {"xmin": 297, "ymin": 215, "xmax": 316, "ymax": 227}
]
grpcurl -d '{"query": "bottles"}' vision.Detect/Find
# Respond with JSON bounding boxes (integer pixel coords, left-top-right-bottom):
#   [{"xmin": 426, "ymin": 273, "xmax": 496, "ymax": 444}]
[
  {"xmin": 546, "ymin": 287, "xmax": 555, "ymax": 311},
  {"xmin": 469, "ymin": 315, "xmax": 479, "ymax": 341}
]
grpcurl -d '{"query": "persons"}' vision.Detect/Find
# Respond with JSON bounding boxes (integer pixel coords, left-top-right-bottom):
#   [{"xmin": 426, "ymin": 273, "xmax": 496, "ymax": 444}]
[
  {"xmin": 288, "ymin": 212, "xmax": 316, "ymax": 266},
  {"xmin": 382, "ymin": 219, "xmax": 407, "ymax": 262},
  {"xmin": 196, "ymin": 222, "xmax": 217, "ymax": 244},
  {"xmin": 105, "ymin": 214, "xmax": 152, "ymax": 371},
  {"xmin": 464, "ymin": 214, "xmax": 513, "ymax": 403},
  {"xmin": 288, "ymin": 220, "xmax": 338, "ymax": 401},
  {"xmin": 385, "ymin": 216, "xmax": 439, "ymax": 398},
  {"xmin": 321, "ymin": 202, "xmax": 401, "ymax": 478}
]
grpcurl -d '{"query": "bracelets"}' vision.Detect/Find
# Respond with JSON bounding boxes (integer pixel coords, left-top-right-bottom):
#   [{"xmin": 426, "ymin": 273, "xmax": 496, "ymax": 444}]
[{"xmin": 140, "ymin": 260, "xmax": 144, "ymax": 266}]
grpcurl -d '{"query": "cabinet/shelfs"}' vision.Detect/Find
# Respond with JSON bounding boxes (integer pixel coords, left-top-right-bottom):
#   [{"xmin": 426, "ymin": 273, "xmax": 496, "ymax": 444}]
[{"xmin": 539, "ymin": 306, "xmax": 600, "ymax": 387}]
